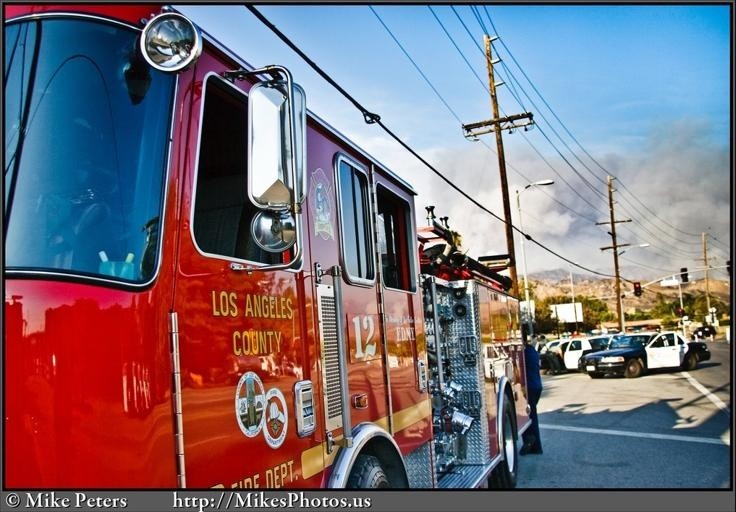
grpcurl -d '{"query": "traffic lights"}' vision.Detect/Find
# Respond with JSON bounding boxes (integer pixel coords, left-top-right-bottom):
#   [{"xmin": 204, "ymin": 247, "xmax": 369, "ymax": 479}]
[
  {"xmin": 678, "ymin": 308, "xmax": 684, "ymax": 317},
  {"xmin": 634, "ymin": 282, "xmax": 642, "ymax": 296}
]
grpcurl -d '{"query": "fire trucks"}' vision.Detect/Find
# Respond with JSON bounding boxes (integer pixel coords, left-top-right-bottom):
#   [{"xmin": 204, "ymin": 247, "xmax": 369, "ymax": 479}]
[{"xmin": 5, "ymin": 5, "xmax": 532, "ymax": 488}]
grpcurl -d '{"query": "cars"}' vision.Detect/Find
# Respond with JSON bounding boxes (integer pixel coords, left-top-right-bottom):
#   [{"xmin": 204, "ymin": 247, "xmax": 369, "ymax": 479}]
[{"xmin": 535, "ymin": 325, "xmax": 718, "ymax": 378}]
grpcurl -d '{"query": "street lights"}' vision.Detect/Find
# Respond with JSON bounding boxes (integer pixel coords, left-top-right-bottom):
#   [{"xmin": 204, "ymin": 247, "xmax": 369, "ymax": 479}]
[
  {"xmin": 617, "ymin": 243, "xmax": 650, "ymax": 334},
  {"xmin": 517, "ymin": 179, "xmax": 555, "ymax": 348}
]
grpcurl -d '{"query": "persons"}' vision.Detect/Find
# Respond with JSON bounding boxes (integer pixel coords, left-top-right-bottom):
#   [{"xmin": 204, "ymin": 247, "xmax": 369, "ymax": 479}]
[{"xmin": 519, "ymin": 330, "xmax": 542, "ymax": 455}]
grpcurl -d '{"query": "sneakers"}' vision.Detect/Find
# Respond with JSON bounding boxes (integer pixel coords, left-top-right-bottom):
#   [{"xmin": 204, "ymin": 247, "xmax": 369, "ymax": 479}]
[{"xmin": 519, "ymin": 433, "xmax": 544, "ymax": 456}]
